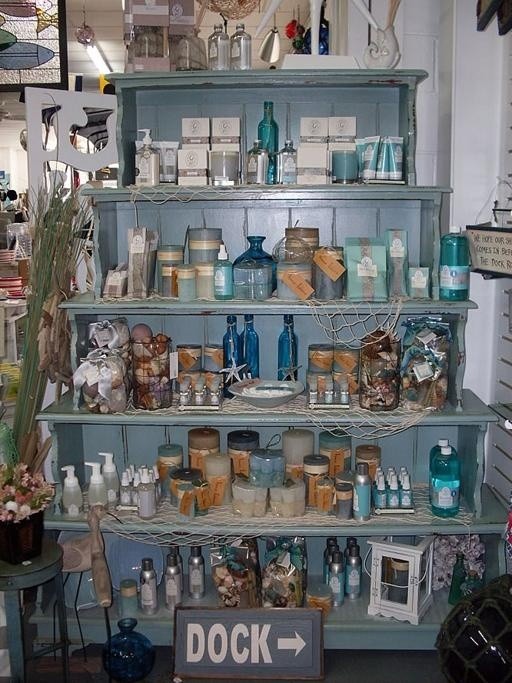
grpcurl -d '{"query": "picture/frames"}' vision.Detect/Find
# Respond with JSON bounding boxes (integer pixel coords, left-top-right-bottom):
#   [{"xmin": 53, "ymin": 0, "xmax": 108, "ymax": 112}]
[{"xmin": 0, "ymin": 0, "xmax": 69, "ymax": 92}]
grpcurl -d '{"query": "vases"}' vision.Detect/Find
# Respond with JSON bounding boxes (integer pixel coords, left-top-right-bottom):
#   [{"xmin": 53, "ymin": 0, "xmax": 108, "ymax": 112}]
[{"xmin": 0, "ymin": 510, "xmax": 45, "ymax": 566}]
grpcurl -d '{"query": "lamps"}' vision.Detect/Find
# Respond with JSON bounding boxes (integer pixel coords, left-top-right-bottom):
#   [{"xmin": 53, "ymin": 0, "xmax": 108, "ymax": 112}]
[{"xmin": 73, "ymin": 6, "xmax": 94, "ymax": 44}]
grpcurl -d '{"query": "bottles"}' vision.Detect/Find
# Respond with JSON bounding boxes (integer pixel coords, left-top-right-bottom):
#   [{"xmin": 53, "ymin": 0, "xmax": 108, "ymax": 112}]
[
  {"xmin": 256, "ymin": 99, "xmax": 279, "ymax": 185},
  {"xmin": 281, "ymin": 139, "xmax": 297, "ymax": 185},
  {"xmin": 238, "ymin": 314, "xmax": 260, "ymax": 379},
  {"xmin": 302, "ymin": 430, "xmax": 459, "ymax": 520},
  {"xmin": 440, "ymin": 232, "xmax": 470, "ymax": 300},
  {"xmin": 249, "ymin": 138, "xmax": 268, "ymax": 186},
  {"xmin": 209, "ymin": 22, "xmax": 229, "ymax": 70},
  {"xmin": 323, "ymin": 537, "xmax": 363, "ymax": 606},
  {"xmin": 230, "ymin": 23, "xmax": 251, "ymax": 69},
  {"xmin": 135, "ymin": 23, "xmax": 164, "ymax": 58},
  {"xmin": 157, "ymin": 224, "xmax": 345, "ymax": 301},
  {"xmin": 117, "ymin": 542, "xmax": 205, "ymax": 615},
  {"xmin": 121, "ymin": 461, "xmax": 164, "ymax": 518},
  {"xmin": 223, "ymin": 317, "xmax": 243, "ymax": 401},
  {"xmin": 176, "ymin": 26, "xmax": 208, "ymax": 71},
  {"xmin": 276, "ymin": 314, "xmax": 301, "ymax": 384}
]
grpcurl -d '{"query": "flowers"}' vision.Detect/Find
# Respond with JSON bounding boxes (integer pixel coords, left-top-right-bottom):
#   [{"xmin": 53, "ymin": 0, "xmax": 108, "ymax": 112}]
[
  {"xmin": 0, "ymin": 461, "xmax": 57, "ymax": 524},
  {"xmin": 285, "ymin": 20, "xmax": 304, "ymax": 51}
]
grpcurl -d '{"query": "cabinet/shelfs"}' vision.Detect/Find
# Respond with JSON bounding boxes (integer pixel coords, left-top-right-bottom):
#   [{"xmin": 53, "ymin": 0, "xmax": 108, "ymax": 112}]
[
  {"xmin": 26, "ymin": 67, "xmax": 511, "ymax": 656},
  {"xmin": 0, "ymin": 539, "xmax": 70, "ymax": 683}
]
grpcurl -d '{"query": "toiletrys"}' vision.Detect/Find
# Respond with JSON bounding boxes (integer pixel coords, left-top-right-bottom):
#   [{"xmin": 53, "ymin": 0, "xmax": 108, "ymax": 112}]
[
  {"xmin": 98, "ymin": 451, "xmax": 120, "ymax": 507},
  {"xmin": 58, "ymin": 465, "xmax": 83, "ymax": 513},
  {"xmin": 116, "ymin": 529, "xmax": 371, "ymax": 624},
  {"xmin": 83, "ymin": 461, "xmax": 109, "ymax": 513}
]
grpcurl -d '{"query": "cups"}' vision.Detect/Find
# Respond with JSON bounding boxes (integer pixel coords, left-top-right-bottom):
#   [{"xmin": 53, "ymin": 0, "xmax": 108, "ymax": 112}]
[{"xmin": 331, "ymin": 149, "xmax": 358, "ymax": 185}]
